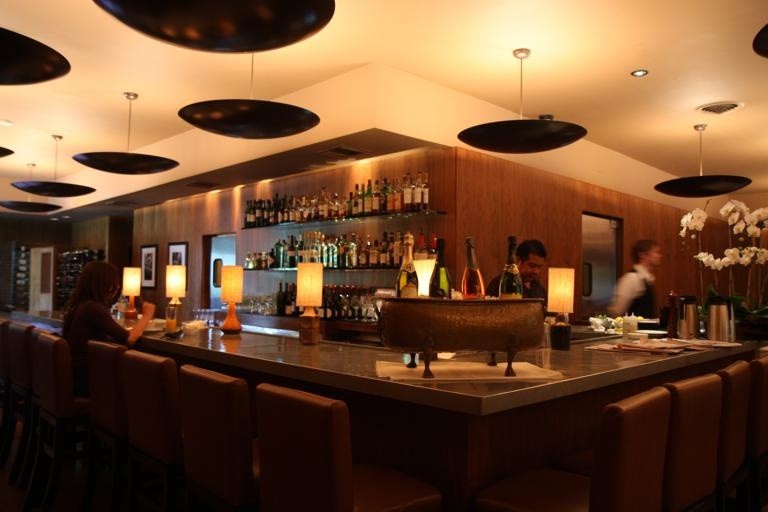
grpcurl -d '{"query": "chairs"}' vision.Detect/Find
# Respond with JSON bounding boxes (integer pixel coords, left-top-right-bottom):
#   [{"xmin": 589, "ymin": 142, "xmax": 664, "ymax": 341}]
[
  {"xmin": 0, "ymin": 320, "xmax": 253, "ymax": 510},
  {"xmin": 667, "ymin": 357, "xmax": 767, "ymax": 512},
  {"xmin": 477, "ymin": 386, "xmax": 675, "ymax": 510},
  {"xmin": 256, "ymin": 383, "xmax": 440, "ymax": 510}
]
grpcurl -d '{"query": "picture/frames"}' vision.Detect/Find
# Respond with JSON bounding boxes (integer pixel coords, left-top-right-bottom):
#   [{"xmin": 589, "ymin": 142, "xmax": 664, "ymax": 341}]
[
  {"xmin": 167, "ymin": 242, "xmax": 187, "ymax": 293},
  {"xmin": 140, "ymin": 245, "xmax": 157, "ymax": 290}
]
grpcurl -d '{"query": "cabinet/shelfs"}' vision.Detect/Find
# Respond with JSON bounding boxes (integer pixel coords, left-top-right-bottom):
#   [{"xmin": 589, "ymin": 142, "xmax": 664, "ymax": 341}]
[{"xmin": 242, "ymin": 208, "xmax": 447, "ymax": 322}]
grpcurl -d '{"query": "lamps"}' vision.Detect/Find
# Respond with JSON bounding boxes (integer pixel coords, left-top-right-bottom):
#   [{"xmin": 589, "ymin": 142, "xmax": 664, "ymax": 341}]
[
  {"xmin": 546, "ymin": 266, "xmax": 576, "ymax": 350},
  {"xmin": 296, "ymin": 263, "xmax": 323, "ymax": 346},
  {"xmin": 122, "ymin": 267, "xmax": 141, "ymax": 318},
  {"xmin": 413, "ymin": 259, "xmax": 437, "ymax": 297},
  {"xmin": 221, "ymin": 266, "xmax": 243, "ymax": 334},
  {"xmin": 164, "ymin": 264, "xmax": 186, "ymax": 326}
]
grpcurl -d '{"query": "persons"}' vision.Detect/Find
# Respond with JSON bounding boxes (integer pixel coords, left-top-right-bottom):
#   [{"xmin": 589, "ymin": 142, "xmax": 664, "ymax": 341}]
[
  {"xmin": 61, "ymin": 261, "xmax": 156, "ymax": 395},
  {"xmin": 174, "ymin": 254, "xmax": 180, "ymax": 263},
  {"xmin": 606, "ymin": 239, "xmax": 664, "ymax": 317},
  {"xmin": 483, "ymin": 240, "xmax": 546, "ymax": 319}
]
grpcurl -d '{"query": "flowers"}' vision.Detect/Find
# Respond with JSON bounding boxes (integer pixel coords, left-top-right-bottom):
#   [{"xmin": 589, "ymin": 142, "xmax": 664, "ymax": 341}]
[{"xmin": 679, "ymin": 200, "xmax": 768, "ymax": 271}]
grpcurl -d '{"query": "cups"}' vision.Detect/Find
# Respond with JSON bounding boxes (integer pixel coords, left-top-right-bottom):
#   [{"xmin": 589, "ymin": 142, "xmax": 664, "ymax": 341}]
[
  {"xmin": 606, "ymin": 329, "xmax": 615, "ymax": 334},
  {"xmin": 549, "ymin": 324, "xmax": 571, "ymax": 351},
  {"xmin": 249, "ymin": 293, "xmax": 276, "ymax": 314},
  {"xmin": 614, "ymin": 322, "xmax": 623, "ymax": 328},
  {"xmin": 164, "ymin": 307, "xmax": 178, "ymax": 332},
  {"xmin": 182, "ymin": 321, "xmax": 199, "ymax": 336}
]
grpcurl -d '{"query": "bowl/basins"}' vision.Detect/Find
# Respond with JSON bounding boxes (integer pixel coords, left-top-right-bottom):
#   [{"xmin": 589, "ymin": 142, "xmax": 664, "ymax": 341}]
[{"xmin": 588, "ymin": 317, "xmax": 611, "ymax": 330}]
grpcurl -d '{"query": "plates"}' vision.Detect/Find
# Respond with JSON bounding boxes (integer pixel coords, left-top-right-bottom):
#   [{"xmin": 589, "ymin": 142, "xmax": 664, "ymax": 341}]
[{"xmin": 140, "ymin": 328, "xmax": 161, "ymax": 333}]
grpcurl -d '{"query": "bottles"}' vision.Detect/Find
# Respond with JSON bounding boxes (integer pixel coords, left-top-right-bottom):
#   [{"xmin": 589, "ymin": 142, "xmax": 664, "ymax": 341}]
[
  {"xmin": 497, "ymin": 235, "xmax": 522, "ymax": 299},
  {"xmin": 623, "ymin": 312, "xmax": 637, "ymax": 336},
  {"xmin": 116, "ymin": 295, "xmax": 129, "ymax": 319},
  {"xmin": 394, "ymin": 233, "xmax": 418, "ymax": 298},
  {"xmin": 460, "ymin": 237, "xmax": 485, "ymax": 299},
  {"xmin": 244, "ymin": 171, "xmax": 431, "ymax": 228},
  {"xmin": 428, "ymin": 238, "xmax": 451, "ymax": 299},
  {"xmin": 277, "ymin": 281, "xmax": 379, "ymax": 322},
  {"xmin": 244, "ymin": 230, "xmax": 437, "ymax": 271}
]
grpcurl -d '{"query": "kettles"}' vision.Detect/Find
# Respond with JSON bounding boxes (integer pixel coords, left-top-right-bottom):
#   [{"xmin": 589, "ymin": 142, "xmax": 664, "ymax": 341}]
[
  {"xmin": 704, "ymin": 295, "xmax": 736, "ymax": 343},
  {"xmin": 676, "ymin": 295, "xmax": 700, "ymax": 340}
]
